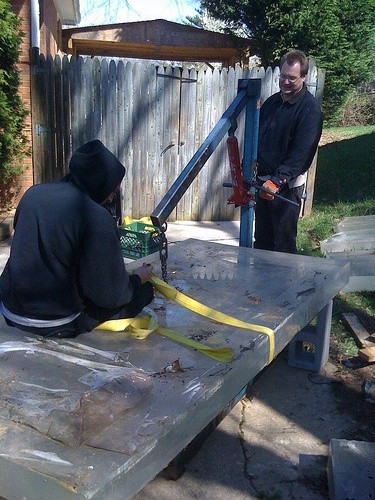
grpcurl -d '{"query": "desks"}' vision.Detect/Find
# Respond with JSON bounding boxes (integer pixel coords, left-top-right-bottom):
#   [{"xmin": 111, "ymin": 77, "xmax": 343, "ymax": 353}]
[{"xmin": 0, "ymin": 237, "xmax": 351, "ymax": 500}]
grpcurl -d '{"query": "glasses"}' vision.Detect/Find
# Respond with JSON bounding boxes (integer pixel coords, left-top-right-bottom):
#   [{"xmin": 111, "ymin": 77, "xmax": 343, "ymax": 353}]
[{"xmin": 278, "ymin": 73, "xmax": 300, "ymax": 83}]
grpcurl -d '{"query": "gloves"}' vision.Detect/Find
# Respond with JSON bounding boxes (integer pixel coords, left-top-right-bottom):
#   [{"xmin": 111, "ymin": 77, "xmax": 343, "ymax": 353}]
[{"xmin": 259, "ymin": 180, "xmax": 278, "ymax": 200}]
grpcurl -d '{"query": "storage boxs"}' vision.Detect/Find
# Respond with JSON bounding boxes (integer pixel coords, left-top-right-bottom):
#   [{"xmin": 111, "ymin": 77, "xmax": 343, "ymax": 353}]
[{"xmin": 117, "ymin": 221, "xmax": 161, "ymax": 260}]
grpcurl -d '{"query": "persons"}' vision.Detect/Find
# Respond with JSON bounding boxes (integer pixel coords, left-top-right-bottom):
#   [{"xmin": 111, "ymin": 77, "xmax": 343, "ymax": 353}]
[
  {"xmin": 0, "ymin": 140, "xmax": 154, "ymax": 339},
  {"xmin": 241, "ymin": 51, "xmax": 323, "ymax": 255}
]
